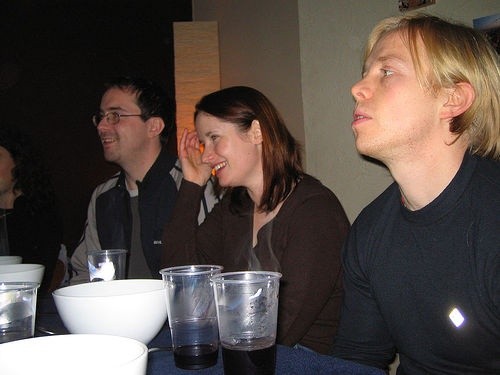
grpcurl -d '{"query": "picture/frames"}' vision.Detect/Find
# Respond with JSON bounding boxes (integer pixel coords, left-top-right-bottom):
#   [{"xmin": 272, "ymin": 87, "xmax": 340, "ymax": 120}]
[{"xmin": 398, "ymin": 0, "xmax": 436, "ymax": 12}]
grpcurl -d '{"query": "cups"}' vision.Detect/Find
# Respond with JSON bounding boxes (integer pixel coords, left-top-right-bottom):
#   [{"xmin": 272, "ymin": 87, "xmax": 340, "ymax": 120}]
[
  {"xmin": 0, "ymin": 281, "xmax": 40, "ymax": 343},
  {"xmin": 212, "ymin": 271, "xmax": 281, "ymax": 375},
  {"xmin": 159, "ymin": 265, "xmax": 224, "ymax": 370},
  {"xmin": 86, "ymin": 249, "xmax": 128, "ymax": 281}
]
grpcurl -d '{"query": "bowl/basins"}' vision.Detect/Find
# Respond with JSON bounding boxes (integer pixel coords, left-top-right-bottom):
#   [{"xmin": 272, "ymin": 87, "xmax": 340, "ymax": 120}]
[
  {"xmin": 52, "ymin": 278, "xmax": 176, "ymax": 346},
  {"xmin": 0, "ymin": 256, "xmax": 22, "ymax": 265},
  {"xmin": 0, "ymin": 264, "xmax": 45, "ymax": 290},
  {"xmin": 0, "ymin": 334, "xmax": 149, "ymax": 375}
]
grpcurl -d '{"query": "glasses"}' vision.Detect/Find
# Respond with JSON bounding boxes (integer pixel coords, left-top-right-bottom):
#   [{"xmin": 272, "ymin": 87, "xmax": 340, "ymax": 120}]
[{"xmin": 92, "ymin": 112, "xmax": 157, "ymax": 125}]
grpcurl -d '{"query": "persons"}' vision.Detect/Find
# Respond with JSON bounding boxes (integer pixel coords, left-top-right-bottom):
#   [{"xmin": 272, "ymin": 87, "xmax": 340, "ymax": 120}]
[
  {"xmin": 159, "ymin": 86, "xmax": 350, "ymax": 355},
  {"xmin": 68, "ymin": 66, "xmax": 228, "ymax": 290},
  {"xmin": 328, "ymin": 12, "xmax": 500, "ymax": 374},
  {"xmin": 0, "ymin": 124, "xmax": 62, "ymax": 296}
]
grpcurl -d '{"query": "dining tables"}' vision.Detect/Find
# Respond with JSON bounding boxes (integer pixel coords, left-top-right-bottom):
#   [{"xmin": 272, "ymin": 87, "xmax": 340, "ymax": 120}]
[{"xmin": 33, "ymin": 309, "xmax": 389, "ymax": 375}]
